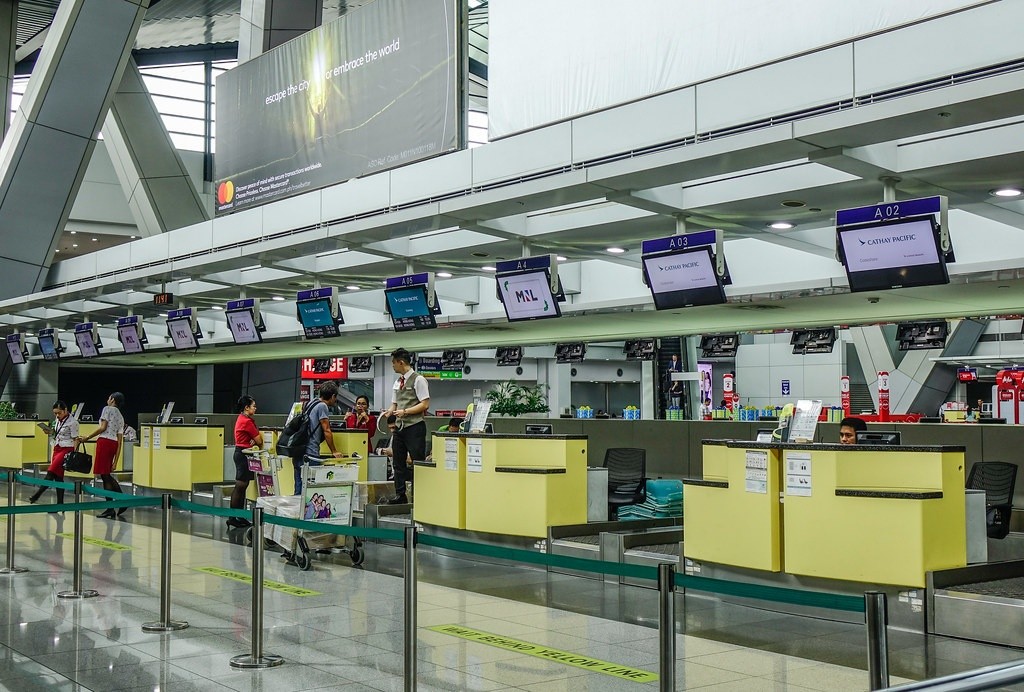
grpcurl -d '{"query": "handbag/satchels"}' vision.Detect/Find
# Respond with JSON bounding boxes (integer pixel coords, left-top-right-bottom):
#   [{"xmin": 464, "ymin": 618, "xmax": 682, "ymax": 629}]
[{"xmin": 64, "ymin": 441, "xmax": 92, "ymax": 474}]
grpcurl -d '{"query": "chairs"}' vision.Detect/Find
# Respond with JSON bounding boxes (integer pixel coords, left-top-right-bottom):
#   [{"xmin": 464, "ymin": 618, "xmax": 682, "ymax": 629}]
[
  {"xmin": 966, "ymin": 462, "xmax": 1019, "ymax": 539},
  {"xmin": 603, "ymin": 448, "xmax": 647, "ymax": 520}
]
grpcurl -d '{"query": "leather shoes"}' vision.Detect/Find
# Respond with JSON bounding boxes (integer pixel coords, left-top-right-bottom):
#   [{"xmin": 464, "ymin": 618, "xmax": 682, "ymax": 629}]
[{"xmin": 389, "ymin": 493, "xmax": 408, "ymax": 503}]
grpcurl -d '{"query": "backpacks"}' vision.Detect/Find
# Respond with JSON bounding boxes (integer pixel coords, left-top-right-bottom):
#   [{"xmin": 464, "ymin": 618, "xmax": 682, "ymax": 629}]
[{"xmin": 276, "ymin": 399, "xmax": 325, "ymax": 456}]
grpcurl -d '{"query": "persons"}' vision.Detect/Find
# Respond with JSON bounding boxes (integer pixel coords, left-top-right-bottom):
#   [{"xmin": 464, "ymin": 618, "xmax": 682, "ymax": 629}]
[
  {"xmin": 700, "ymin": 370, "xmax": 711, "ymax": 403},
  {"xmin": 123, "ymin": 421, "xmax": 137, "ymax": 442},
  {"xmin": 226, "ymin": 395, "xmax": 263, "ymax": 528},
  {"xmin": 293, "ymin": 380, "xmax": 342, "ymax": 496},
  {"xmin": 76, "ymin": 392, "xmax": 129, "ymax": 518},
  {"xmin": 382, "ymin": 348, "xmax": 430, "ymax": 505},
  {"xmin": 29, "ymin": 400, "xmax": 80, "ymax": 514},
  {"xmin": 666, "ymin": 353, "xmax": 684, "ymax": 407},
  {"xmin": 305, "ymin": 493, "xmax": 331, "ymax": 519},
  {"xmin": 840, "ymin": 417, "xmax": 868, "ymax": 445},
  {"xmin": 344, "ymin": 395, "xmax": 464, "ymax": 480},
  {"xmin": 700, "ymin": 399, "xmax": 727, "ymax": 420}
]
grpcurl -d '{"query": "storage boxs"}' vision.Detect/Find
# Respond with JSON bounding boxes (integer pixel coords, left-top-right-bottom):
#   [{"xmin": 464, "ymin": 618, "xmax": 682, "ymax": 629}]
[{"xmin": 255, "ymin": 495, "xmax": 348, "ymax": 558}]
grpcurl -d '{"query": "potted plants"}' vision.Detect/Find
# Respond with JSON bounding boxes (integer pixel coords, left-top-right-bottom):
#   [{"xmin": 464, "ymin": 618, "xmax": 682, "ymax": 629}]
[{"xmin": 486, "ymin": 380, "xmax": 551, "ymax": 417}]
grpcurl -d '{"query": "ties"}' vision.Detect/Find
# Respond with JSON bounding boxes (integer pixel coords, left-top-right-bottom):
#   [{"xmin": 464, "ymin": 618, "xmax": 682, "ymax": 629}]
[{"xmin": 399, "ymin": 375, "xmax": 405, "ymax": 389}]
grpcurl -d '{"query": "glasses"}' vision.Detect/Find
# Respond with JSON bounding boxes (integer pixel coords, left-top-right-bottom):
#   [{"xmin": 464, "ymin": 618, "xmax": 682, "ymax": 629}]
[{"xmin": 356, "ymin": 403, "xmax": 366, "ymax": 407}]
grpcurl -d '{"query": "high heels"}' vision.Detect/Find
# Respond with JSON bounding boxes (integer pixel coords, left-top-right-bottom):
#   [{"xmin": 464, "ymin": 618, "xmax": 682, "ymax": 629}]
[
  {"xmin": 48, "ymin": 511, "xmax": 65, "ymax": 513},
  {"xmin": 29, "ymin": 495, "xmax": 35, "ymax": 504},
  {"xmin": 226, "ymin": 519, "xmax": 247, "ymax": 530},
  {"xmin": 96, "ymin": 510, "xmax": 116, "ymax": 519},
  {"xmin": 117, "ymin": 507, "xmax": 127, "ymax": 516}
]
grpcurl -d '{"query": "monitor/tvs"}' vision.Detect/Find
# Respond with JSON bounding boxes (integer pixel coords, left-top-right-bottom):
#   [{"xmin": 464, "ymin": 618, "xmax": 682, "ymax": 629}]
[
  {"xmin": 756, "ymin": 428, "xmax": 781, "ymax": 441},
  {"xmin": 5, "ymin": 341, "xmax": 27, "ymax": 365},
  {"xmin": 73, "ymin": 330, "xmax": 101, "ymax": 359},
  {"xmin": 854, "ymin": 430, "xmax": 902, "ymax": 444},
  {"xmin": 170, "ymin": 416, "xmax": 185, "ymax": 424},
  {"xmin": 494, "ymin": 267, "xmax": 562, "ymax": 324},
  {"xmin": 525, "ymin": 423, "xmax": 553, "ymax": 434},
  {"xmin": 329, "ymin": 419, "xmax": 348, "ymax": 428},
  {"xmin": 640, "ymin": 244, "xmax": 727, "ymax": 311},
  {"xmin": 310, "ymin": 320, "xmax": 948, "ymax": 373},
  {"xmin": 193, "ymin": 417, "xmax": 209, "ymax": 424},
  {"xmin": 835, "ymin": 213, "xmax": 950, "ymax": 293},
  {"xmin": 16, "ymin": 412, "xmax": 40, "ymax": 419},
  {"xmin": 484, "ymin": 422, "xmax": 493, "ymax": 433},
  {"xmin": 384, "ymin": 284, "xmax": 437, "ymax": 333},
  {"xmin": 37, "ymin": 335, "xmax": 61, "ymax": 362},
  {"xmin": 295, "ymin": 297, "xmax": 340, "ymax": 340},
  {"xmin": 165, "ymin": 316, "xmax": 200, "ymax": 351},
  {"xmin": 81, "ymin": 414, "xmax": 93, "ymax": 421},
  {"xmin": 224, "ymin": 307, "xmax": 264, "ymax": 345},
  {"xmin": 116, "ymin": 324, "xmax": 145, "ymax": 354}
]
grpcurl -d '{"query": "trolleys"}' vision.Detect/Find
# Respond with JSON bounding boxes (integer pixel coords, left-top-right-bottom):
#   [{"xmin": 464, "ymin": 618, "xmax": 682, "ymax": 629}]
[{"xmin": 246, "ymin": 451, "xmax": 364, "ymax": 571}]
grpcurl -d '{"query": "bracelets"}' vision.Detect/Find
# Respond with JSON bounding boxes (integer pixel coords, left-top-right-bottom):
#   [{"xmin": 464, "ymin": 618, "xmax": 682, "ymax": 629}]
[
  {"xmin": 87, "ymin": 435, "xmax": 91, "ymax": 439},
  {"xmin": 403, "ymin": 409, "xmax": 407, "ymax": 417}
]
